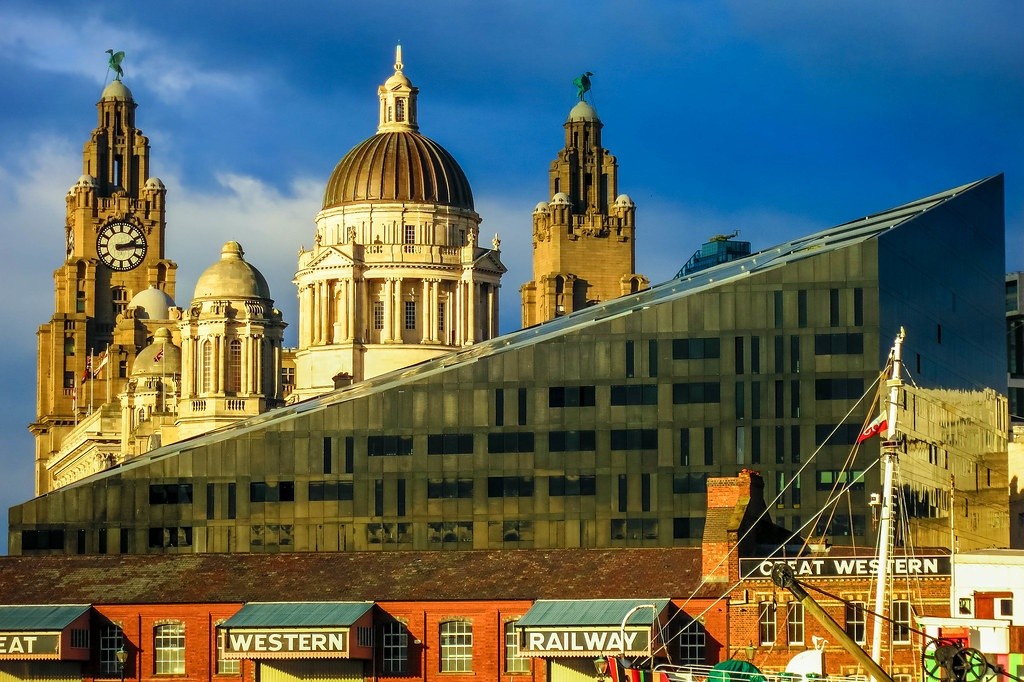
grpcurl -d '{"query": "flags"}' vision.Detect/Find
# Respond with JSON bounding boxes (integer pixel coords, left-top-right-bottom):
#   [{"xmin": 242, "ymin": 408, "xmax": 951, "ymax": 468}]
[
  {"xmin": 80, "ymin": 356, "xmax": 92, "ymax": 385},
  {"xmin": 72, "ymin": 382, "xmax": 78, "ymax": 412},
  {"xmin": 93, "ymin": 349, "xmax": 109, "ymax": 376},
  {"xmin": 857, "ymin": 410, "xmax": 888, "ymax": 443},
  {"xmin": 153, "ymin": 346, "xmax": 165, "ymax": 363}
]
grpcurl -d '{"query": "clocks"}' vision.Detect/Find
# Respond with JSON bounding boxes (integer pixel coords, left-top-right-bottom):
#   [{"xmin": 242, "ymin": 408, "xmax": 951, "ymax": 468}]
[{"xmin": 96, "ymin": 221, "xmax": 147, "ymax": 272}]
